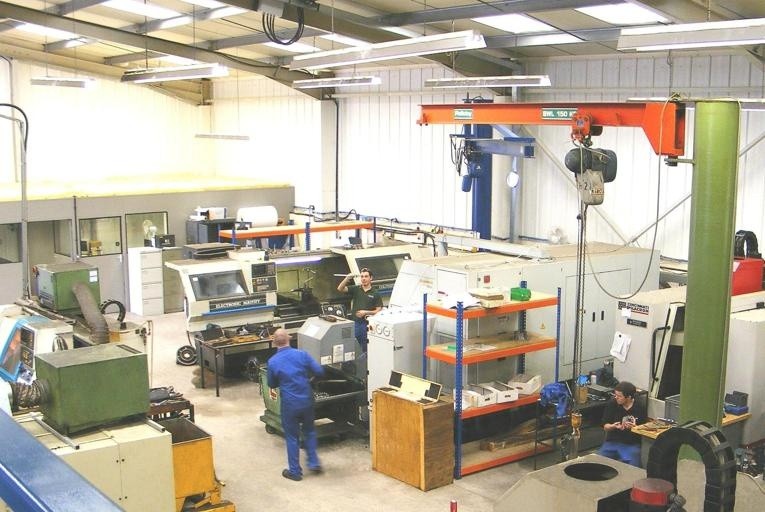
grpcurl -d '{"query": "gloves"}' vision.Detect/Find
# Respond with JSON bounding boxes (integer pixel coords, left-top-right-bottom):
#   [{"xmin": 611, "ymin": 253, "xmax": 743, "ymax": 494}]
[{"xmin": 346, "ymin": 273, "xmax": 354, "ymax": 282}]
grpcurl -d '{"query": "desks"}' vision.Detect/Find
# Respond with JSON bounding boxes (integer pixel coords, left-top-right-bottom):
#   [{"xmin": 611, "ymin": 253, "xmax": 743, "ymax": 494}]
[{"xmin": 631, "ymin": 410, "xmax": 753, "ymax": 470}]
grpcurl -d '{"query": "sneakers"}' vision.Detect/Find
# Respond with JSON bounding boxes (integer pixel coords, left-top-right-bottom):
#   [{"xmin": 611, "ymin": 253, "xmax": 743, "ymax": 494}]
[
  {"xmin": 283, "ymin": 469, "xmax": 303, "ymax": 480},
  {"xmin": 309, "ymin": 465, "xmax": 321, "ymax": 472}
]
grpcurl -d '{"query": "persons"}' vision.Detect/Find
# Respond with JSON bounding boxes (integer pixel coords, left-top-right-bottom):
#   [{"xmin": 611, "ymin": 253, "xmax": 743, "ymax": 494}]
[
  {"xmin": 598, "ymin": 382, "xmax": 641, "ymax": 467},
  {"xmin": 336, "ymin": 267, "xmax": 383, "ymax": 358},
  {"xmin": 266, "ymin": 328, "xmax": 324, "ymax": 481}
]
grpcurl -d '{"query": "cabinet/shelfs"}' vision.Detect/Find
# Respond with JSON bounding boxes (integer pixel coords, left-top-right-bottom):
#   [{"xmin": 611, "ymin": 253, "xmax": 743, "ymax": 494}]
[
  {"xmin": 162, "ymin": 246, "xmax": 184, "ymax": 315},
  {"xmin": 370, "ymin": 386, "xmax": 455, "ymax": 491},
  {"xmin": 0, "ymin": 411, "xmax": 176, "ymax": 511},
  {"xmin": 421, "ymin": 280, "xmax": 562, "ymax": 477},
  {"xmin": 217, "ymin": 213, "xmax": 374, "ymax": 253},
  {"xmin": 128, "ymin": 245, "xmax": 165, "ymax": 316}
]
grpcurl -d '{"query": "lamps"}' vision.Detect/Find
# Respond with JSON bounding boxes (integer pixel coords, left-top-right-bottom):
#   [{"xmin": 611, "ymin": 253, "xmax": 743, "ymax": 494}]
[
  {"xmin": 292, "ymin": 33, "xmax": 382, "ymax": 90},
  {"xmin": 29, "ymin": 0, "xmax": 96, "ymax": 90},
  {"xmin": 615, "ymin": 1, "xmax": 765, "ymax": 52},
  {"xmin": 194, "ymin": 68, "xmax": 250, "ymax": 141},
  {"xmin": 289, "ymin": 1, "xmax": 485, "ymax": 72},
  {"xmin": 423, "ymin": 20, "xmax": 552, "ymax": 90},
  {"xmin": 119, "ymin": 1, "xmax": 230, "ymax": 84}
]
